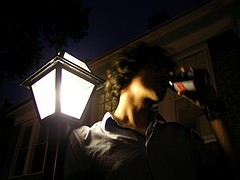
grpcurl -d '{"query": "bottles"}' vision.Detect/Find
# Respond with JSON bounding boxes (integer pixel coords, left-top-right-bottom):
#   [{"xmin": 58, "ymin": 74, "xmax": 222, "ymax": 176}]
[{"xmin": 156, "ymin": 68, "xmax": 211, "ymax": 96}]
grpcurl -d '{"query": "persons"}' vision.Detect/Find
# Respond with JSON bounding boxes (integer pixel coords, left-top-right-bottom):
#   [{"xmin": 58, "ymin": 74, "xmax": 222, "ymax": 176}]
[{"xmin": 62, "ymin": 43, "xmax": 211, "ymax": 180}]
